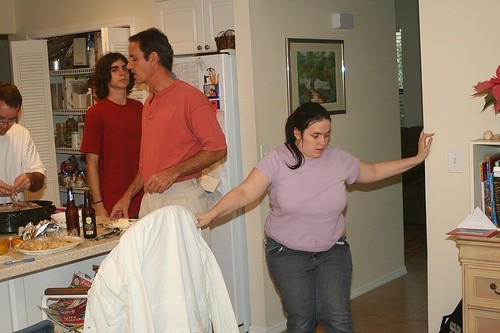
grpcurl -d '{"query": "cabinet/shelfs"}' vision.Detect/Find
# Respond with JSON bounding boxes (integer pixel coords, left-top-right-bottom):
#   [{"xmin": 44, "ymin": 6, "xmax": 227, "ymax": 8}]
[
  {"xmin": 157, "ymin": 0, "xmax": 235, "ymax": 55},
  {"xmin": 11, "ymin": 27, "xmax": 130, "ymax": 206},
  {"xmin": 455, "ymin": 236, "xmax": 500, "ymax": 333}
]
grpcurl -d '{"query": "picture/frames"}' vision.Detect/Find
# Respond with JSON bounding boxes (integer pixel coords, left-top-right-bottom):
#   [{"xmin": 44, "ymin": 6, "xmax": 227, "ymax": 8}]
[{"xmin": 285, "ymin": 38, "xmax": 346, "ymax": 116}]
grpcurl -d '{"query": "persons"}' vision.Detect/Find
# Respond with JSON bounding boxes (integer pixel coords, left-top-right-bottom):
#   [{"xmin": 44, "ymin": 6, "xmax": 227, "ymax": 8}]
[
  {"xmin": 195, "ymin": 101, "xmax": 434, "ymax": 333},
  {"xmin": 110, "ymin": 28, "xmax": 227, "ymax": 246},
  {"xmin": 80, "ymin": 54, "xmax": 143, "ymax": 219},
  {"xmin": 0, "ymin": 82, "xmax": 46, "ymax": 204}
]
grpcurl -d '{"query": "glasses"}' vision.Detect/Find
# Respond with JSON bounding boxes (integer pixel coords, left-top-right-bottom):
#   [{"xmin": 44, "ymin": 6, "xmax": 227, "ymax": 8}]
[{"xmin": 0, "ymin": 116, "xmax": 18, "ymax": 123}]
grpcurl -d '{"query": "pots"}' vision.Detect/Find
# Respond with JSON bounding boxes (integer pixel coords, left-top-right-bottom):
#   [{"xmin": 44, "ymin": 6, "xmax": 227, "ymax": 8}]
[{"xmin": 0, "ymin": 200, "xmax": 53, "ymax": 234}]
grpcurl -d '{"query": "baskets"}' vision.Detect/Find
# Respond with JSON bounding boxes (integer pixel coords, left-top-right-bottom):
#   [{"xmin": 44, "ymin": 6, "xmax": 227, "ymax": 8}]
[{"xmin": 215, "ymin": 30, "xmax": 235, "ymax": 53}]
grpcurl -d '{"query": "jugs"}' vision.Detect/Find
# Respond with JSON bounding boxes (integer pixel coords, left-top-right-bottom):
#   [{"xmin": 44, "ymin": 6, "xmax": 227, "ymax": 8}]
[{"xmin": 70, "ymin": 82, "xmax": 89, "ymax": 109}]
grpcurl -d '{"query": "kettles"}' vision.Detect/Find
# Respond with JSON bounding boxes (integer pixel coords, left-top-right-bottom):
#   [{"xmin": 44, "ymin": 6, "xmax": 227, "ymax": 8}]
[{"xmin": 50, "ymin": 56, "xmax": 63, "ymax": 71}]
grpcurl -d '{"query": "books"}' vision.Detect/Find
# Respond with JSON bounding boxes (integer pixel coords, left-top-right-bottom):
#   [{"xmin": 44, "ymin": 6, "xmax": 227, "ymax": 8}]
[{"xmin": 480, "ymin": 153, "xmax": 500, "ymax": 228}]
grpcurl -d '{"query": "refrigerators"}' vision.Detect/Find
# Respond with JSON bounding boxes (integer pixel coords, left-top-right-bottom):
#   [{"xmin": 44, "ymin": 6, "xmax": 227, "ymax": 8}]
[{"xmin": 171, "ymin": 49, "xmax": 250, "ymax": 333}]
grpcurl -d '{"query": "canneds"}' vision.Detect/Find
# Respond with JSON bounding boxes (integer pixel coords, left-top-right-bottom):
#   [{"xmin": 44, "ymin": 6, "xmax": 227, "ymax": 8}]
[
  {"xmin": 75, "ymin": 174, "xmax": 85, "ymax": 188},
  {"xmin": 65, "ymin": 131, "xmax": 79, "ymax": 148}
]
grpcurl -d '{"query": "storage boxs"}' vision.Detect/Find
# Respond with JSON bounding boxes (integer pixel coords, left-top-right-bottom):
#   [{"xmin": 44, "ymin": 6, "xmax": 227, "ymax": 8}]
[{"xmin": 72, "ymin": 93, "xmax": 87, "ymax": 108}]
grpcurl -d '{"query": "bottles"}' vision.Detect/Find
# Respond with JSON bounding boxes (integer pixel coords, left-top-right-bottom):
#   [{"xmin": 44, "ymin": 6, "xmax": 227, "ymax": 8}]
[
  {"xmin": 82, "ymin": 191, "xmax": 96, "ymax": 239},
  {"xmin": 65, "ymin": 186, "xmax": 80, "ymax": 236},
  {"xmin": 78, "ymin": 116, "xmax": 85, "ymax": 146},
  {"xmin": 64, "ymin": 115, "xmax": 78, "ymax": 148}
]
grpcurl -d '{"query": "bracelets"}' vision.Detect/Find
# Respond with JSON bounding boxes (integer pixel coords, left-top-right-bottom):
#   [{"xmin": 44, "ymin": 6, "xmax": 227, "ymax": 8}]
[{"xmin": 93, "ymin": 200, "xmax": 103, "ymax": 204}]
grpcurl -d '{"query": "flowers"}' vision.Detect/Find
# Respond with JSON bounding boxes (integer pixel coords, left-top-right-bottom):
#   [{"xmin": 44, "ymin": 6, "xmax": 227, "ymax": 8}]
[{"xmin": 472, "ymin": 66, "xmax": 500, "ymax": 116}]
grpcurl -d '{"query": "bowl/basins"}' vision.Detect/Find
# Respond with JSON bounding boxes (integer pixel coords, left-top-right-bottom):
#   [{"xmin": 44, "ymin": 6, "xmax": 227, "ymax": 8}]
[{"xmin": 51, "ymin": 212, "xmax": 83, "ymax": 229}]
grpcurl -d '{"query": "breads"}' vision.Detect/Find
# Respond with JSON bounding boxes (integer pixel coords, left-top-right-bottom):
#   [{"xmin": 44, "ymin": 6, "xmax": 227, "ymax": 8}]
[{"xmin": 0, "ymin": 237, "xmax": 25, "ymax": 255}]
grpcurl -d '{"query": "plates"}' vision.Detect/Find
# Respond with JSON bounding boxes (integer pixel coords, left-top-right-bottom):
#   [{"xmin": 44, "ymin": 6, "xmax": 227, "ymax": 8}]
[
  {"xmin": 14, "ymin": 236, "xmax": 82, "ymax": 255},
  {"xmin": 103, "ymin": 219, "xmax": 139, "ymax": 232}
]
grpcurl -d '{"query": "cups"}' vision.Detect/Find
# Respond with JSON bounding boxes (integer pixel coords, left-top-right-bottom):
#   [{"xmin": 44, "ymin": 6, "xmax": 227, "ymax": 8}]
[{"xmin": 50, "ymin": 83, "xmax": 62, "ymax": 110}]
